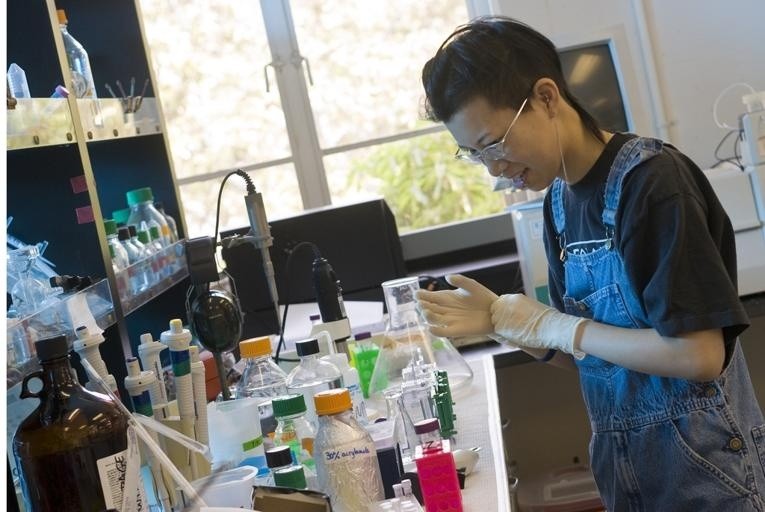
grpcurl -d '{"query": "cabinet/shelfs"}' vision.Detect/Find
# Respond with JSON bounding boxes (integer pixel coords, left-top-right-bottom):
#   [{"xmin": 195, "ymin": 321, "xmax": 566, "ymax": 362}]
[{"xmin": 7, "ymin": 0, "xmax": 191, "ymax": 393}]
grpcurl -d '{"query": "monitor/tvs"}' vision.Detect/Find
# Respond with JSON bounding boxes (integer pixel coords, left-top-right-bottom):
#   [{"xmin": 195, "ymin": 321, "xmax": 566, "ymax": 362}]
[{"xmin": 489, "ymin": 36, "xmax": 635, "ymax": 211}]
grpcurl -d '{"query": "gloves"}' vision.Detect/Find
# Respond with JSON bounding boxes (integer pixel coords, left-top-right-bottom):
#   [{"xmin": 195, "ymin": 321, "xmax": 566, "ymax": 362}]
[
  {"xmin": 491, "ymin": 293, "xmax": 589, "ymax": 361},
  {"xmin": 412, "ymin": 274, "xmax": 499, "ymax": 336}
]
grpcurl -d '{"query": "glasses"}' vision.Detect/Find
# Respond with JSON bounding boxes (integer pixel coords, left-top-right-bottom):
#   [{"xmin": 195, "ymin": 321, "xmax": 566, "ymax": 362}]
[{"xmin": 454, "ymin": 101, "xmax": 527, "ymax": 164}]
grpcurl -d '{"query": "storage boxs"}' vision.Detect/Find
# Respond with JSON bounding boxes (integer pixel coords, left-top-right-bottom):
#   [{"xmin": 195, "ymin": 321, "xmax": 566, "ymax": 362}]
[{"xmin": 515, "ymin": 466, "xmax": 605, "ymax": 511}]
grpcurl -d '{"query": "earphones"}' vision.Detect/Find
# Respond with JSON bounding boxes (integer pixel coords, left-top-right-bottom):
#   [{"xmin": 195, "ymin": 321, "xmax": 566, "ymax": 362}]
[{"xmin": 542, "ymin": 93, "xmax": 550, "ymax": 103}]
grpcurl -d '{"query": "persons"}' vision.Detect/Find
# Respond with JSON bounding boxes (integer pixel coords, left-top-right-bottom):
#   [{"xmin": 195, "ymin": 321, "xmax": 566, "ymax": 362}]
[{"xmin": 413, "ymin": 16, "xmax": 765, "ymax": 511}]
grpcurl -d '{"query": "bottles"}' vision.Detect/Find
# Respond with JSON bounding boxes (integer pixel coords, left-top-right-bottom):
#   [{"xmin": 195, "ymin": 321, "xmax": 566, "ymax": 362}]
[
  {"xmin": 7, "ymin": 63, "xmax": 31, "ymax": 98},
  {"xmin": 12, "ymin": 334, "xmax": 150, "ymax": 512},
  {"xmin": 235, "ymin": 276, "xmax": 473, "ymax": 512},
  {"xmin": 104, "ymin": 186, "xmax": 186, "ymax": 303},
  {"xmin": 57, "ymin": 9, "xmax": 98, "ymax": 98}
]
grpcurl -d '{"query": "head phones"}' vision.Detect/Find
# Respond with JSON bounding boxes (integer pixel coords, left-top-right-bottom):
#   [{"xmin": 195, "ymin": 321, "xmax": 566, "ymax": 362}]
[{"xmin": 185, "ymin": 236, "xmax": 246, "ymax": 354}]
